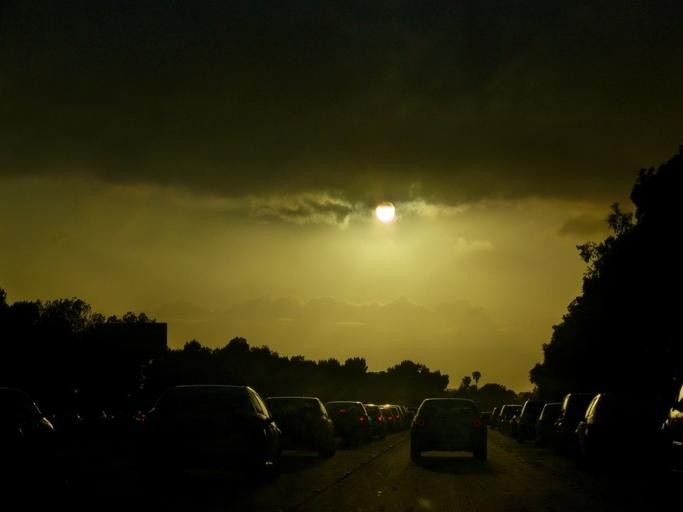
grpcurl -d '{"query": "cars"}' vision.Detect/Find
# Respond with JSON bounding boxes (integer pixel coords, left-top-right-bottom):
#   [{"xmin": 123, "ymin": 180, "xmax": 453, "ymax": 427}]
[
  {"xmin": 490, "ymin": 392, "xmax": 682, "ymax": 471},
  {"xmin": 139, "ymin": 384, "xmax": 418, "ymax": 482},
  {"xmin": 410, "ymin": 397, "xmax": 487, "ymax": 463}
]
